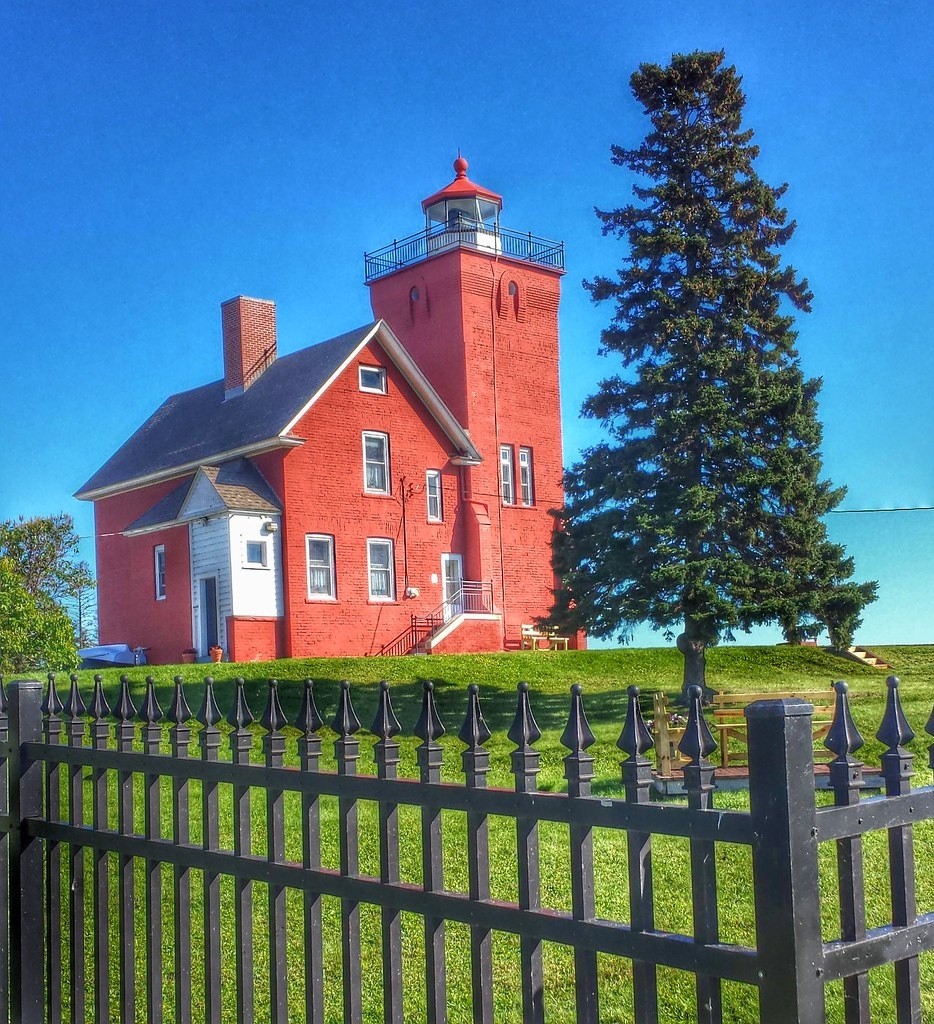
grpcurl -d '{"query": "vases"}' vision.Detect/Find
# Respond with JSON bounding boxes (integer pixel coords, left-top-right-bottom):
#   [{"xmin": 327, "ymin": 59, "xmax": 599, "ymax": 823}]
[
  {"xmin": 182, "ymin": 654, "xmax": 196, "ymax": 663},
  {"xmin": 209, "ymin": 650, "xmax": 223, "ymax": 662}
]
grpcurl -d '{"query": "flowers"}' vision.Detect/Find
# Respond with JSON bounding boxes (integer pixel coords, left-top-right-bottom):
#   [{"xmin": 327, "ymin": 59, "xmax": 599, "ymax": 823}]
[
  {"xmin": 209, "ymin": 644, "xmax": 221, "ymax": 650},
  {"xmin": 182, "ymin": 648, "xmax": 197, "ymax": 654}
]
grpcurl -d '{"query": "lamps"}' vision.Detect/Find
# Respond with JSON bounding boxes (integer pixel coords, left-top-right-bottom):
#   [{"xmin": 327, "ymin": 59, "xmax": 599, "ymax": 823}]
[{"xmin": 265, "ymin": 521, "xmax": 279, "ymax": 532}]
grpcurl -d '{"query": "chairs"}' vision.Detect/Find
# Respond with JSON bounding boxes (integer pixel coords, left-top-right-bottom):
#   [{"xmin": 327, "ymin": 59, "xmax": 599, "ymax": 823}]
[{"xmin": 652, "ymin": 691, "xmax": 692, "ymax": 776}]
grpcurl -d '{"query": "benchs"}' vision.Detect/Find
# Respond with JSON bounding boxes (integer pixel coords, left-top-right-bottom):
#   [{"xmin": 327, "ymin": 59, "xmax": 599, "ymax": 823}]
[
  {"xmin": 521, "ymin": 623, "xmax": 569, "ymax": 651},
  {"xmin": 712, "ymin": 690, "xmax": 839, "ymax": 768}
]
